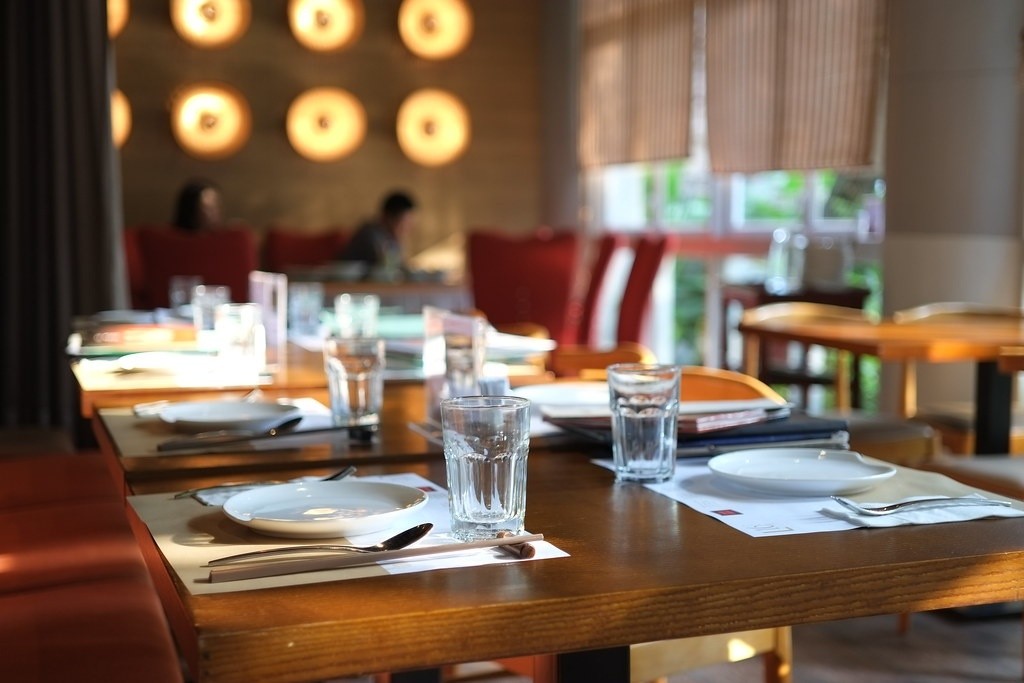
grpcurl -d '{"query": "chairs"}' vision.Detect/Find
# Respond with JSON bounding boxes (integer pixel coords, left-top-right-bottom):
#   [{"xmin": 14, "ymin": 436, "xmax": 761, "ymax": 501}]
[{"xmin": 125, "ymin": 222, "xmax": 875, "ymax": 679}]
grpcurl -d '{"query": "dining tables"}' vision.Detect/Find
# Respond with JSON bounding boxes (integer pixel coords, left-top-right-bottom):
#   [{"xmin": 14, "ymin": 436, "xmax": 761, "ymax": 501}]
[{"xmin": 70, "ymin": 319, "xmax": 1024, "ymax": 683}]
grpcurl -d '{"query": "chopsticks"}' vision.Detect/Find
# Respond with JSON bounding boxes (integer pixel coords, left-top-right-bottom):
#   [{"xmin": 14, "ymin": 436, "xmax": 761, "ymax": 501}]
[{"xmin": 205, "ymin": 535, "xmax": 546, "ymax": 583}]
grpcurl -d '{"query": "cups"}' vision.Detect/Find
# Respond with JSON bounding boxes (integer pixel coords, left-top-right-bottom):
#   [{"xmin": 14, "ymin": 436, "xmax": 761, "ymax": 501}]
[
  {"xmin": 607, "ymin": 360, "xmax": 678, "ymax": 484},
  {"xmin": 189, "ymin": 284, "xmax": 231, "ymax": 336},
  {"xmin": 423, "ymin": 315, "xmax": 483, "ymax": 397},
  {"xmin": 171, "ymin": 274, "xmax": 204, "ymax": 317},
  {"xmin": 324, "ymin": 336, "xmax": 385, "ymax": 428},
  {"xmin": 440, "ymin": 396, "xmax": 531, "ymax": 540},
  {"xmin": 328, "ymin": 290, "xmax": 383, "ymax": 355}
]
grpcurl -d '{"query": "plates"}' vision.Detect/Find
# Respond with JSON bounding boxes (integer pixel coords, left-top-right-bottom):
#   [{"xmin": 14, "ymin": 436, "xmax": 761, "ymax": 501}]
[
  {"xmin": 366, "ymin": 312, "xmax": 900, "ymax": 499},
  {"xmin": 107, "ymin": 339, "xmax": 430, "ymax": 538}
]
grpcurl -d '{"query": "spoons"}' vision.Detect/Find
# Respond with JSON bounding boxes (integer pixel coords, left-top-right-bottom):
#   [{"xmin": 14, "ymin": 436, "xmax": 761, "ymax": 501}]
[
  {"xmin": 153, "ymin": 413, "xmax": 304, "ymax": 453},
  {"xmin": 208, "ymin": 522, "xmax": 435, "ymax": 568}
]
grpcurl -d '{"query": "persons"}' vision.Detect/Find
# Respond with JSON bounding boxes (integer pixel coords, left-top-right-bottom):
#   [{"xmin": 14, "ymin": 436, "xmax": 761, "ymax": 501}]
[{"xmin": 337, "ymin": 191, "xmax": 443, "ymax": 284}]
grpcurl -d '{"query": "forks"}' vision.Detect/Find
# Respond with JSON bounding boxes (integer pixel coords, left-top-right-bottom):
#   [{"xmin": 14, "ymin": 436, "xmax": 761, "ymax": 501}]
[
  {"xmin": 175, "ymin": 464, "xmax": 356, "ymax": 500},
  {"xmin": 828, "ymin": 493, "xmax": 1011, "ymax": 515}
]
